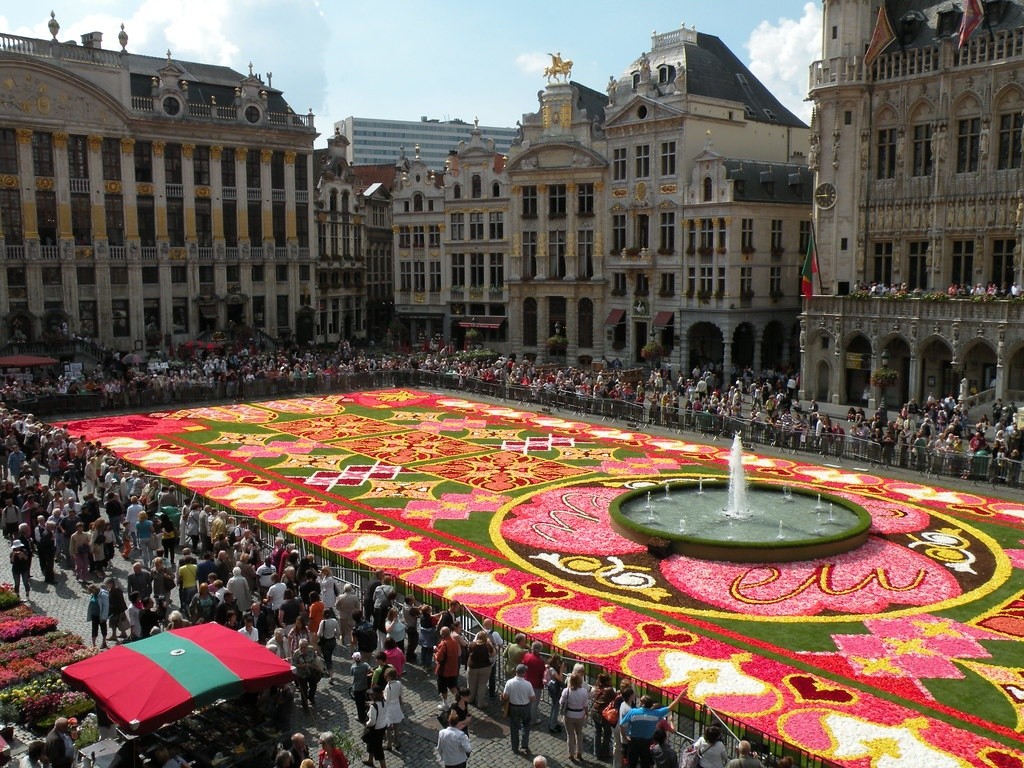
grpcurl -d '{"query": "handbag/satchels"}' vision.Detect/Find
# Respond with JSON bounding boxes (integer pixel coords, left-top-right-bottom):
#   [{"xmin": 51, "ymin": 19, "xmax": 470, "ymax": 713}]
[
  {"xmin": 601, "ymin": 695, "xmax": 622, "ymax": 726},
  {"xmin": 360, "ymin": 726, "xmax": 375, "ymax": 742},
  {"xmin": 437, "ymin": 706, "xmax": 457, "ymax": 728},
  {"xmin": 1, "ymin": 403, "xmax": 339, "ymax": 685},
  {"xmin": 681, "ymin": 743, "xmax": 701, "ymax": 768},
  {"xmin": 559, "ymin": 689, "xmax": 569, "ymax": 715}
]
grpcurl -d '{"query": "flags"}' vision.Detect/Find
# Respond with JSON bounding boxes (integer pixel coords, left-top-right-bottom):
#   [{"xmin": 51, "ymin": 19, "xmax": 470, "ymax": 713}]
[
  {"xmin": 802, "ymin": 224, "xmax": 818, "ymax": 301},
  {"xmin": 958, "ymin": 0, "xmax": 988, "ymax": 50},
  {"xmin": 863, "ymin": 0, "xmax": 897, "ymax": 68}
]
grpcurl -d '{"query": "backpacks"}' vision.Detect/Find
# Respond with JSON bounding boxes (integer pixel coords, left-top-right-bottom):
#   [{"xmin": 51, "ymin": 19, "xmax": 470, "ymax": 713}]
[
  {"xmin": 356, "ymin": 621, "xmax": 377, "ymax": 652},
  {"xmin": 543, "ymin": 665, "xmax": 553, "ymax": 686}
]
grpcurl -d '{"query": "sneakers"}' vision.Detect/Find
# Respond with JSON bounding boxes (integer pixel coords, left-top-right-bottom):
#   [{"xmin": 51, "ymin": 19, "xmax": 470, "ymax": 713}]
[{"xmin": 437, "ymin": 704, "xmax": 449, "ymax": 711}]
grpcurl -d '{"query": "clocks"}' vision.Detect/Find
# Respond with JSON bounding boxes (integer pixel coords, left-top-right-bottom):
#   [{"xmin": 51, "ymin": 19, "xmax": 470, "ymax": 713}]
[{"xmin": 815, "ymin": 182, "xmax": 837, "ymax": 209}]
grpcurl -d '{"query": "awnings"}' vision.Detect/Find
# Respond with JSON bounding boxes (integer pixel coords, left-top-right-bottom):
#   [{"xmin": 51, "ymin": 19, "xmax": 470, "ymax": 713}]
[
  {"xmin": 61, "ymin": 621, "xmax": 296, "ymax": 736},
  {"xmin": 0, "ymin": 355, "xmax": 59, "ymax": 368},
  {"xmin": 459, "ymin": 317, "xmax": 507, "ymax": 328},
  {"xmin": 604, "ymin": 309, "xmax": 626, "ymax": 327},
  {"xmin": 652, "ymin": 312, "xmax": 674, "ymax": 330}
]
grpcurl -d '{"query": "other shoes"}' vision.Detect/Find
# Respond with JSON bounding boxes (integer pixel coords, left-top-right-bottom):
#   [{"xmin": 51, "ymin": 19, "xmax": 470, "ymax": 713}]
[
  {"xmin": 577, "ymin": 753, "xmax": 582, "ymax": 759},
  {"xmin": 549, "ymin": 724, "xmax": 561, "ymax": 733},
  {"xmin": 302, "ymin": 702, "xmax": 309, "ymax": 712},
  {"xmin": 329, "ymin": 677, "xmax": 335, "ymax": 683},
  {"xmin": 355, "ymin": 715, "xmax": 361, "ymax": 720},
  {"xmin": 512, "ymin": 747, "xmax": 518, "ymax": 752},
  {"xmin": 569, "ymin": 756, "xmax": 575, "ymax": 760},
  {"xmin": 307, "ymin": 696, "xmax": 316, "ymax": 703},
  {"xmin": 395, "ymin": 742, "xmax": 400, "ymax": 750},
  {"xmin": 382, "ymin": 745, "xmax": 393, "ymax": 751},
  {"xmin": 362, "ymin": 758, "xmax": 374, "ymax": 766}
]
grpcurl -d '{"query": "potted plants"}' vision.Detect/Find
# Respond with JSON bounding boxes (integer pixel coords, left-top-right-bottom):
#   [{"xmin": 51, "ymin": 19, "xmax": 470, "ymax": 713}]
[{"xmin": 0, "ymin": 704, "xmax": 16, "ymax": 741}]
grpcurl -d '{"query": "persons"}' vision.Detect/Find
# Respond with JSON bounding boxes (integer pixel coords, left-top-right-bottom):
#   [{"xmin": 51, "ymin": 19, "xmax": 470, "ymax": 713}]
[
  {"xmin": 808, "ymin": 121, "xmax": 1024, "ymax": 170},
  {"xmin": 853, "ymin": 279, "xmax": 1024, "ymax": 300},
  {"xmin": 859, "ymin": 198, "xmax": 1024, "ymax": 232},
  {"xmin": 855, "ymin": 238, "xmax": 1021, "ymax": 273},
  {"xmin": 606, "ymin": 52, "xmax": 685, "ymax": 108},
  {"xmin": 548, "ymin": 51, "xmax": 563, "ymax": 73},
  {"xmin": 359, "ymin": 190, "xmax": 365, "ymax": 207},
  {"xmin": 29, "ymin": 239, "xmax": 298, "ymax": 263},
  {"xmin": 0, "ymin": 330, "xmax": 1024, "ymax": 768}
]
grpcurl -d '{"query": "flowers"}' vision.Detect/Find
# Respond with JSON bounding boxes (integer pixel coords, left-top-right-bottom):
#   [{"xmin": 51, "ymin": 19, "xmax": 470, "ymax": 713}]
[{"xmin": 0, "ymin": 582, "xmax": 104, "ymax": 723}]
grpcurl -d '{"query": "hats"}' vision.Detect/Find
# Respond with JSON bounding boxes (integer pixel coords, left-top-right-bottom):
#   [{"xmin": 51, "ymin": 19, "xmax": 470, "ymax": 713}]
[{"xmin": 352, "ymin": 652, "xmax": 361, "ymax": 661}]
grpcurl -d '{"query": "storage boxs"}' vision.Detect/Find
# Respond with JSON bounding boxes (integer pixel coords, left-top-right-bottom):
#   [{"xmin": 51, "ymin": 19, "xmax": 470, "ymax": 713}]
[{"xmin": 111, "ymin": 697, "xmax": 292, "ymax": 768}]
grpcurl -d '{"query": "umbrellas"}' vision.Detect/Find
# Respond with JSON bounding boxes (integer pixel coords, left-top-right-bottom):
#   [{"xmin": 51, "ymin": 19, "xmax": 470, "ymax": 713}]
[
  {"xmin": 122, "ymin": 353, "xmax": 149, "ymax": 367},
  {"xmin": 182, "ymin": 340, "xmax": 206, "ymax": 355},
  {"xmin": 201, "ymin": 341, "xmax": 224, "ymax": 353}
]
